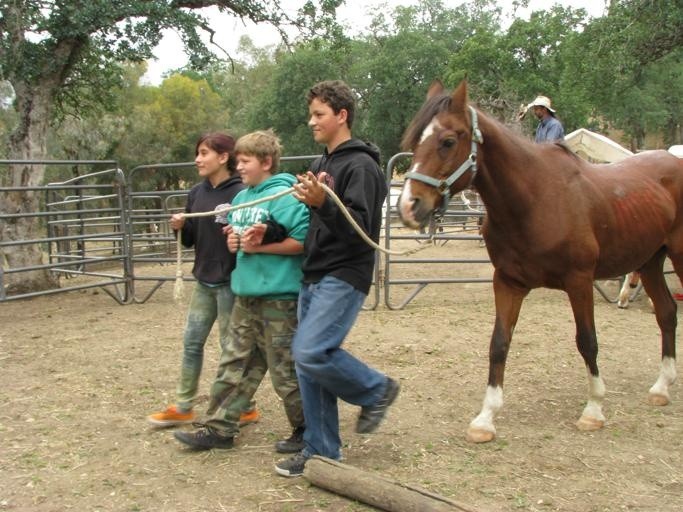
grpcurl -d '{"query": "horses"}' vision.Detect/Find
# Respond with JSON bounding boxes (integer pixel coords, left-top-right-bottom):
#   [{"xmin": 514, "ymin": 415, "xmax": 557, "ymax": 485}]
[{"xmin": 395, "ymin": 72, "xmax": 683, "ymax": 445}]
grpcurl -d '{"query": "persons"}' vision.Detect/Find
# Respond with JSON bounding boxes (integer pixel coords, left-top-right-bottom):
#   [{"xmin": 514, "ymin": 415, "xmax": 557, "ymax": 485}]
[
  {"xmin": 271, "ymin": 82, "xmax": 401, "ymax": 479},
  {"xmin": 142, "ymin": 134, "xmax": 263, "ymax": 427},
  {"xmin": 523, "ymin": 94, "xmax": 564, "ymax": 145},
  {"xmin": 170, "ymin": 127, "xmax": 309, "ymax": 453}
]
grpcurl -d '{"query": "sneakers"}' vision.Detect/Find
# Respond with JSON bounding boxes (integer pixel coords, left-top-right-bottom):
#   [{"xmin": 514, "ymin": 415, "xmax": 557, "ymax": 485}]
[
  {"xmin": 273, "ymin": 451, "xmax": 343, "ymax": 478},
  {"xmin": 147, "ymin": 403, "xmax": 197, "ymax": 428},
  {"xmin": 237, "ymin": 410, "xmax": 261, "ymax": 427},
  {"xmin": 274, "ymin": 425, "xmax": 307, "ymax": 453},
  {"xmin": 173, "ymin": 424, "xmax": 232, "ymax": 449},
  {"xmin": 355, "ymin": 375, "xmax": 402, "ymax": 435}
]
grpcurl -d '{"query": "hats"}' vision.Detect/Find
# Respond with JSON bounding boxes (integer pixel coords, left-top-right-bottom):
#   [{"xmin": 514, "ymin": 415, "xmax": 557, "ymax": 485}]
[{"xmin": 524, "ymin": 95, "xmax": 556, "ymax": 114}]
